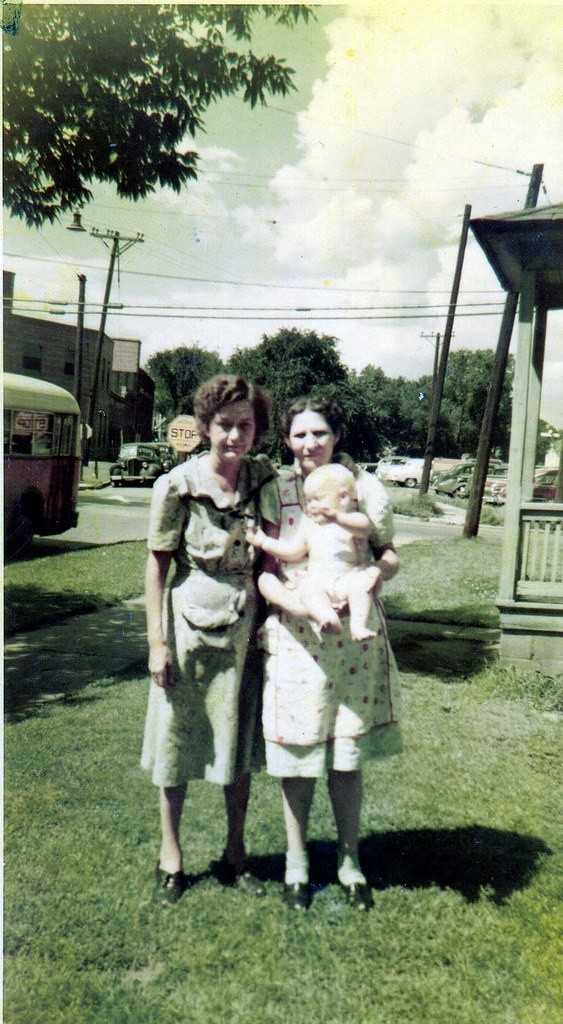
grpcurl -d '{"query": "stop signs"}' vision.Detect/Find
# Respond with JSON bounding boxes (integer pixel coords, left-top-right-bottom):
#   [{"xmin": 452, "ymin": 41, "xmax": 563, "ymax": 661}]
[{"xmin": 167, "ymin": 416, "xmax": 202, "ymax": 452}]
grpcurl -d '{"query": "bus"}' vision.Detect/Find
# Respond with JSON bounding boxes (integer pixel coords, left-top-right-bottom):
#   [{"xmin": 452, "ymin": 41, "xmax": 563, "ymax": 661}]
[{"xmin": 3, "ymin": 373, "xmax": 83, "ymax": 562}]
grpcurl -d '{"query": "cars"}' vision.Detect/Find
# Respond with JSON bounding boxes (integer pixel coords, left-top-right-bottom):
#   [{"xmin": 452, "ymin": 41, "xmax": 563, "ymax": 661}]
[
  {"xmin": 108, "ymin": 442, "xmax": 174, "ymax": 487},
  {"xmin": 355, "ymin": 454, "xmax": 562, "ymax": 506}
]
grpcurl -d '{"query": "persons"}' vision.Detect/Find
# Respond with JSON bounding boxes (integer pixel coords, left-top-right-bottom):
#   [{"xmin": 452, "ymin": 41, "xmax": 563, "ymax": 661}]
[
  {"xmin": 140, "ymin": 373, "xmax": 358, "ymax": 903},
  {"xmin": 245, "ymin": 464, "xmax": 377, "ymax": 641},
  {"xmin": 258, "ymin": 394, "xmax": 401, "ymax": 912}
]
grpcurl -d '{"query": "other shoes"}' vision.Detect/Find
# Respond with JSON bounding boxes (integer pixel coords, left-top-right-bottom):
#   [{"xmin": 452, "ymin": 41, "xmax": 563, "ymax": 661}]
[
  {"xmin": 155, "ymin": 858, "xmax": 185, "ymax": 903},
  {"xmin": 342, "ymin": 883, "xmax": 375, "ymax": 912},
  {"xmin": 233, "ymin": 870, "xmax": 267, "ymax": 898},
  {"xmin": 282, "ymin": 879, "xmax": 313, "ymax": 913}
]
